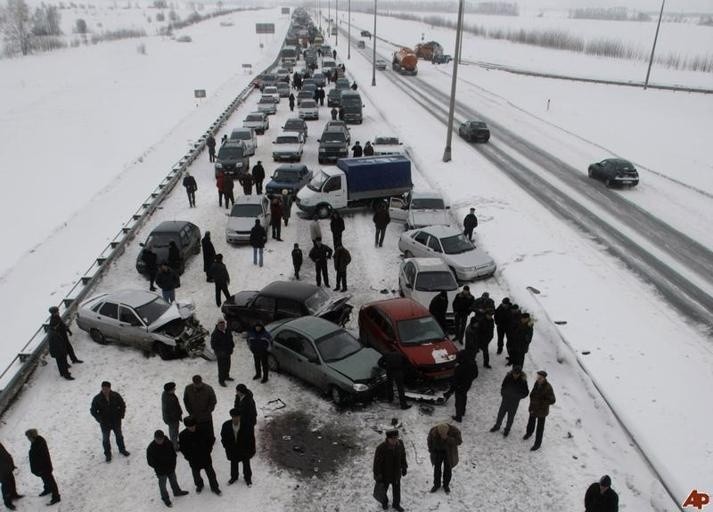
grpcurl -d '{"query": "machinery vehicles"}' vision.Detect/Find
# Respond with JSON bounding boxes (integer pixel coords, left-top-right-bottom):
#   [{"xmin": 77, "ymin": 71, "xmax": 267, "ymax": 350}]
[
  {"xmin": 416, "ymin": 41, "xmax": 442, "ymax": 62},
  {"xmin": 392, "ymin": 48, "xmax": 418, "ymax": 76}
]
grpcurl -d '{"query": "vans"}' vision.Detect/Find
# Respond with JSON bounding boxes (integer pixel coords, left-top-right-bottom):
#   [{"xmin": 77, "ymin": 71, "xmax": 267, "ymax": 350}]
[{"xmin": 323, "ymin": 56, "xmax": 335, "ymax": 72}]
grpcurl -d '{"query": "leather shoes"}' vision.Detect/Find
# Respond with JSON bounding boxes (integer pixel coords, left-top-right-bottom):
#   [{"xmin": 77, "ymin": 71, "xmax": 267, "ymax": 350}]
[
  {"xmin": 261, "ymin": 378, "xmax": 267, "ymax": 383},
  {"xmin": 174, "ymin": 491, "xmax": 188, "ymax": 497},
  {"xmin": 326, "ymin": 284, "xmax": 329, "ymax": 288},
  {"xmin": 504, "ymin": 430, "xmax": 508, "ymax": 437},
  {"xmin": 484, "ymin": 364, "xmax": 491, "ymax": 368},
  {"xmin": 73, "ymin": 360, "xmax": 83, "ymax": 364},
  {"xmin": 67, "ymin": 364, "xmax": 71, "ymax": 368},
  {"xmin": 150, "ymin": 288, "xmax": 155, "ymax": 291},
  {"xmin": 531, "ymin": 444, "xmax": 540, "ymax": 450},
  {"xmin": 225, "ymin": 378, "xmax": 233, "ymax": 381},
  {"xmin": 165, "ymin": 501, "xmax": 172, "ymax": 506},
  {"xmin": 334, "ymin": 288, "xmax": 340, "ymax": 291},
  {"xmin": 153, "ymin": 287, "xmax": 156, "ymax": 289},
  {"xmin": 39, "ymin": 489, "xmax": 52, "ymax": 496},
  {"xmin": 443, "ymin": 484, "xmax": 450, "ymax": 493},
  {"xmin": 341, "ymin": 289, "xmax": 347, "ymax": 292},
  {"xmin": 7, "ymin": 504, "xmax": 15, "ymax": 510},
  {"xmin": 228, "ymin": 477, "xmax": 238, "ymax": 484},
  {"xmin": 383, "ymin": 504, "xmax": 387, "ymax": 510},
  {"xmin": 490, "ymin": 424, "xmax": 500, "ymax": 432},
  {"xmin": 452, "ymin": 415, "xmax": 461, "ymax": 421},
  {"xmin": 106, "ymin": 456, "xmax": 111, "ymax": 461},
  {"xmin": 220, "ymin": 381, "xmax": 226, "ymax": 387},
  {"xmin": 253, "ymin": 375, "xmax": 260, "ymax": 379},
  {"xmin": 247, "ymin": 480, "xmax": 252, "ymax": 487},
  {"xmin": 66, "ymin": 377, "xmax": 74, "ymax": 380},
  {"xmin": 120, "ymin": 450, "xmax": 129, "ymax": 456},
  {"xmin": 391, "ymin": 504, "xmax": 404, "ymax": 512},
  {"xmin": 196, "ymin": 483, "xmax": 204, "ymax": 492},
  {"xmin": 214, "ymin": 488, "xmax": 221, "ymax": 495},
  {"xmin": 431, "ymin": 485, "xmax": 441, "ymax": 492},
  {"xmin": 523, "ymin": 433, "xmax": 532, "ymax": 440},
  {"xmin": 13, "ymin": 495, "xmax": 22, "ymax": 499},
  {"xmin": 402, "ymin": 404, "xmax": 412, "ymax": 409},
  {"xmin": 47, "ymin": 496, "xmax": 60, "ymax": 505}
]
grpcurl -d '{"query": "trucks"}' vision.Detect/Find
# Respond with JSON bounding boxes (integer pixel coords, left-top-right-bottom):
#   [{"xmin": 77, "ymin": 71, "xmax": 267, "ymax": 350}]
[{"xmin": 296, "ymin": 156, "xmax": 412, "ymax": 219}]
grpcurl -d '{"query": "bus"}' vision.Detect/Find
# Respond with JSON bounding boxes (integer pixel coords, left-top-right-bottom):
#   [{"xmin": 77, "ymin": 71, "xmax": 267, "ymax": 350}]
[
  {"xmin": 341, "ymin": 90, "xmax": 365, "ymax": 124},
  {"xmin": 282, "ymin": 45, "xmax": 297, "ymax": 65}
]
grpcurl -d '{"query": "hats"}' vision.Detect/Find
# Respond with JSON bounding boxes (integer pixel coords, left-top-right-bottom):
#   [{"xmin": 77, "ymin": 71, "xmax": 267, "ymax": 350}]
[
  {"xmin": 510, "ymin": 304, "xmax": 518, "ymax": 309},
  {"xmin": 600, "ymin": 474, "xmax": 611, "ymax": 487},
  {"xmin": 502, "ymin": 297, "xmax": 509, "ymax": 304},
  {"xmin": 537, "ymin": 370, "xmax": 547, "ymax": 376},
  {"xmin": 386, "ymin": 429, "xmax": 399, "ymax": 438},
  {"xmin": 184, "ymin": 416, "xmax": 198, "ymax": 426},
  {"xmin": 463, "ymin": 285, "xmax": 469, "ymax": 290},
  {"xmin": 230, "ymin": 409, "xmax": 240, "ymax": 416},
  {"xmin": 512, "ymin": 366, "xmax": 521, "ymax": 373},
  {"xmin": 154, "ymin": 430, "xmax": 164, "ymax": 438},
  {"xmin": 164, "ymin": 382, "xmax": 175, "ymax": 390},
  {"xmin": 521, "ymin": 312, "xmax": 530, "ymax": 318},
  {"xmin": 438, "ymin": 423, "xmax": 448, "ymax": 438},
  {"xmin": 236, "ymin": 384, "xmax": 246, "ymax": 391},
  {"xmin": 102, "ymin": 382, "xmax": 110, "ymax": 387},
  {"xmin": 471, "ymin": 317, "xmax": 478, "ymax": 323}
]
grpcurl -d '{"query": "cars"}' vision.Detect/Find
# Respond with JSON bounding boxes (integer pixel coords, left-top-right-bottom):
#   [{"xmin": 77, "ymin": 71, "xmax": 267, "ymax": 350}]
[
  {"xmin": 324, "ymin": 120, "xmax": 351, "ymax": 145},
  {"xmin": 387, "ymin": 191, "xmax": 451, "ymax": 229},
  {"xmin": 459, "ymin": 121, "xmax": 490, "ymax": 142},
  {"xmin": 135, "ymin": 221, "xmax": 201, "ymax": 277},
  {"xmin": 263, "ymin": 315, "xmax": 387, "ymax": 405},
  {"xmin": 332, "ymin": 27, "xmax": 338, "ymax": 35},
  {"xmin": 281, "ymin": 119, "xmax": 308, "ymax": 143},
  {"xmin": 298, "ymin": 78, "xmax": 316, "ymax": 98},
  {"xmin": 222, "ymin": 281, "xmax": 353, "ymax": 332},
  {"xmin": 76, "ymin": 290, "xmax": 209, "ymax": 360},
  {"xmin": 272, "ymin": 132, "xmax": 304, "ymax": 161},
  {"xmin": 216, "ymin": 140, "xmax": 249, "ymax": 178},
  {"xmin": 336, "ymin": 79, "xmax": 348, "ymax": 89},
  {"xmin": 398, "ymin": 257, "xmax": 460, "ymax": 327},
  {"xmin": 277, "ymin": 82, "xmax": 290, "ymax": 97},
  {"xmin": 276, "ymin": 68, "xmax": 290, "ymax": 81},
  {"xmin": 398, "ymin": 224, "xmax": 497, "ymax": 282},
  {"xmin": 243, "ymin": 112, "xmax": 269, "ymax": 133},
  {"xmin": 328, "ymin": 89, "xmax": 340, "ymax": 105},
  {"xmin": 376, "ymin": 60, "xmax": 387, "ymax": 71},
  {"xmin": 258, "ymin": 95, "xmax": 276, "ymax": 115},
  {"xmin": 317, "ymin": 133, "xmax": 348, "ymax": 164},
  {"xmin": 263, "ymin": 86, "xmax": 280, "ymax": 102},
  {"xmin": 358, "ymin": 297, "xmax": 459, "ymax": 389},
  {"xmin": 225, "ymin": 195, "xmax": 271, "ymax": 244},
  {"xmin": 228, "ymin": 127, "xmax": 257, "ymax": 157},
  {"xmin": 587, "ymin": 159, "xmax": 639, "ymax": 187},
  {"xmin": 265, "ymin": 164, "xmax": 313, "ymax": 201},
  {"xmin": 313, "ymin": 74, "xmax": 326, "ymax": 86},
  {"xmin": 298, "ymin": 98, "xmax": 319, "ymax": 119},
  {"xmin": 255, "ymin": 74, "xmax": 277, "ymax": 88}
]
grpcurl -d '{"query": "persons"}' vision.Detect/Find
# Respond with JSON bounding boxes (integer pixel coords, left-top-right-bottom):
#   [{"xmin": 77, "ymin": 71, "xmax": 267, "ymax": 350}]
[
  {"xmin": 426, "ymin": 423, "xmax": 462, "ymax": 495},
  {"xmin": 352, "ymin": 140, "xmax": 362, "ymax": 156},
  {"xmin": 584, "ymin": 475, "xmax": 620, "ymax": 512},
  {"xmin": 222, "ymin": 135, "xmax": 229, "ymax": 143},
  {"xmin": 331, "ymin": 107, "xmax": 338, "ymax": 121},
  {"xmin": 480, "ymin": 312, "xmax": 493, "ymax": 369},
  {"xmin": 315, "ymin": 86, "xmax": 325, "ymax": 106},
  {"xmin": 429, "ymin": 290, "xmax": 448, "ymax": 337},
  {"xmin": 49, "ymin": 307, "xmax": 83, "ymax": 366},
  {"xmin": 510, "ymin": 313, "xmax": 533, "ymax": 371},
  {"xmin": 494, "ymin": 297, "xmax": 513, "ymax": 355},
  {"xmin": 373, "ymin": 202, "xmax": 391, "ymax": 248},
  {"xmin": 465, "ymin": 317, "xmax": 480, "ymax": 361},
  {"xmin": 282, "ymin": 190, "xmax": 291, "ymax": 226},
  {"xmin": 364, "ymin": 142, "xmax": 374, "ymax": 156},
  {"xmin": 489, "ymin": 366, "xmax": 530, "ymax": 437},
  {"xmin": 253, "ymin": 161, "xmax": 265, "ymax": 194},
  {"xmin": 332, "ymin": 241, "xmax": 351, "ymax": 292},
  {"xmin": 143, "ymin": 244, "xmax": 158, "ymax": 292},
  {"xmin": 211, "ymin": 318, "xmax": 234, "ymax": 388},
  {"xmin": 523, "ymin": 371, "xmax": 556, "ymax": 451},
  {"xmin": 90, "ymin": 381, "xmax": 129, "ymax": 462},
  {"xmin": 372, "ymin": 429, "xmax": 408, "ymax": 512},
  {"xmin": 47, "ymin": 318, "xmax": 75, "ymax": 381},
  {"xmin": 246, "ymin": 321, "xmax": 273, "ymax": 384},
  {"xmin": 179, "ymin": 417, "xmax": 222, "ymax": 496},
  {"xmin": 167, "ymin": 241, "xmax": 182, "ymax": 276},
  {"xmin": 504, "ymin": 304, "xmax": 523, "ymax": 366},
  {"xmin": 223, "ymin": 172, "xmax": 236, "ymax": 209},
  {"xmin": 26, "ymin": 429, "xmax": 61, "ymax": 506},
  {"xmin": 234, "ymin": 384, "xmax": 257, "ymax": 429},
  {"xmin": 161, "ymin": 382, "xmax": 184, "ymax": 453},
  {"xmin": 216, "ymin": 168, "xmax": 226, "ymax": 205},
  {"xmin": 220, "ymin": 409, "xmax": 256, "ymax": 486},
  {"xmin": 250, "ymin": 219, "xmax": 267, "ymax": 267},
  {"xmin": 0, "ymin": 443, "xmax": 25, "ymax": 510},
  {"xmin": 182, "ymin": 171, "xmax": 198, "ymax": 208},
  {"xmin": 309, "ymin": 237, "xmax": 333, "ymax": 288},
  {"xmin": 451, "ymin": 286, "xmax": 475, "ymax": 345},
  {"xmin": 378, "ymin": 344, "xmax": 412, "ymax": 411},
  {"xmin": 147, "ymin": 431, "xmax": 189, "ymax": 507},
  {"xmin": 240, "ymin": 169, "xmax": 253, "ymax": 195},
  {"xmin": 310, "ymin": 215, "xmax": 323, "ymax": 243},
  {"xmin": 201, "ymin": 231, "xmax": 216, "ymax": 272},
  {"xmin": 183, "ymin": 376, "xmax": 217, "ymax": 443},
  {"xmin": 206, "ymin": 254, "xmax": 233, "ymax": 307},
  {"xmin": 269, "ymin": 199, "xmax": 283, "ymax": 241},
  {"xmin": 444, "ymin": 350, "xmax": 479, "ymax": 422},
  {"xmin": 207, "ymin": 132, "xmax": 216, "ymax": 163},
  {"xmin": 292, "ymin": 243, "xmax": 303, "ymax": 280},
  {"xmin": 289, "ymin": 93, "xmax": 296, "ymax": 111},
  {"xmin": 474, "ymin": 292, "xmax": 495, "ymax": 319},
  {"xmin": 155, "ymin": 260, "xmax": 181, "ymax": 304},
  {"xmin": 330, "ymin": 212, "xmax": 345, "ymax": 247},
  {"xmin": 285, "ymin": 48, "xmax": 358, "ymax": 90},
  {"xmin": 463, "ymin": 208, "xmax": 477, "ymax": 241}
]
